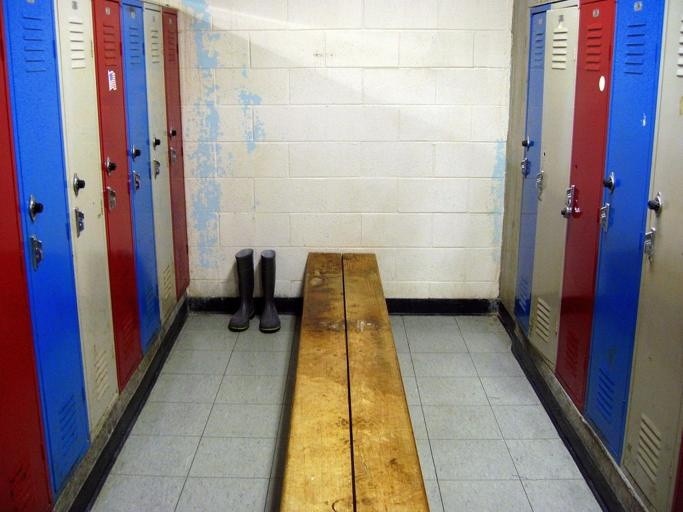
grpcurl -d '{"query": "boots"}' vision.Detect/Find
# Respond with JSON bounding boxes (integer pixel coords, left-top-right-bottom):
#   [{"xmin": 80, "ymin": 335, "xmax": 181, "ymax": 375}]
[
  {"xmin": 229, "ymin": 249, "xmax": 255, "ymax": 331},
  {"xmin": 260, "ymin": 250, "xmax": 280, "ymax": 331}
]
